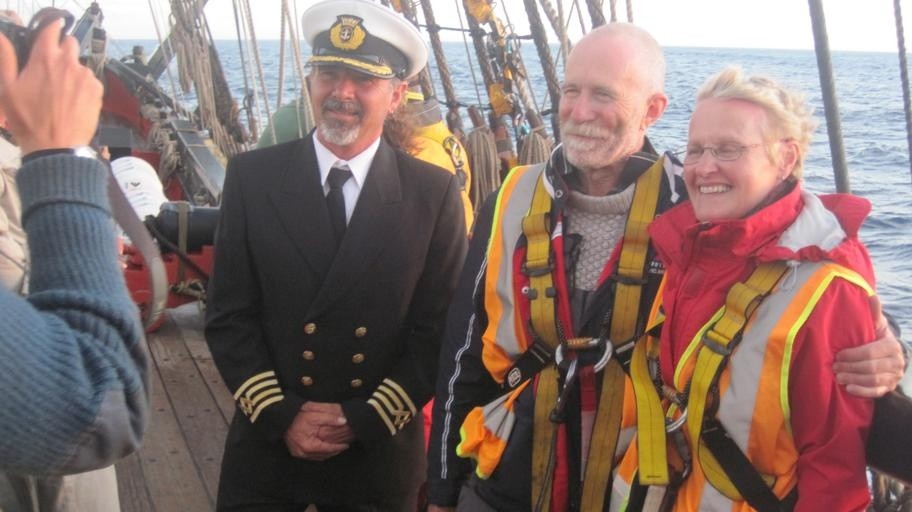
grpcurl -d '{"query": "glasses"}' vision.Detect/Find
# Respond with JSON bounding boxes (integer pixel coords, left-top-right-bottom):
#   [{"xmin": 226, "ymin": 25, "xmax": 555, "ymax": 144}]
[{"xmin": 674, "ymin": 142, "xmax": 764, "ymax": 164}]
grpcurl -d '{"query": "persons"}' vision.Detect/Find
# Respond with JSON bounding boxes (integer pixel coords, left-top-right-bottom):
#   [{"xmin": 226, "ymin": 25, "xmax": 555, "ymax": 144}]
[
  {"xmin": 383, "ymin": 74, "xmax": 474, "ymax": 240},
  {"xmin": 1, "ymin": 6, "xmax": 154, "ymax": 512},
  {"xmin": 202, "ymin": 0, "xmax": 469, "ymax": 511},
  {"xmin": 255, "ymin": 73, "xmax": 318, "ymax": 149},
  {"xmin": 608, "ymin": 64, "xmax": 877, "ymax": 512},
  {"xmin": 421, "ymin": 21, "xmax": 910, "ymax": 512}
]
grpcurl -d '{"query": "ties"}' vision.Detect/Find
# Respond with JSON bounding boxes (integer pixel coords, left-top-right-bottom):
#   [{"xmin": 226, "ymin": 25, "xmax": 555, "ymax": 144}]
[{"xmin": 325, "ymin": 167, "xmax": 351, "ymax": 231}]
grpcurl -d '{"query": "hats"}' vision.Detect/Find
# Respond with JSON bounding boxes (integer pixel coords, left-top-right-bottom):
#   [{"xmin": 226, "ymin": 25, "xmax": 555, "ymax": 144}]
[{"xmin": 301, "ymin": 0, "xmax": 428, "ymax": 80}]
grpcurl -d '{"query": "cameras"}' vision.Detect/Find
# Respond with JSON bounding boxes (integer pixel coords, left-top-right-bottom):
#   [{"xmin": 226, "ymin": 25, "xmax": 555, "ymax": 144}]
[{"xmin": 0, "ymin": 19, "xmax": 47, "ymax": 149}]
